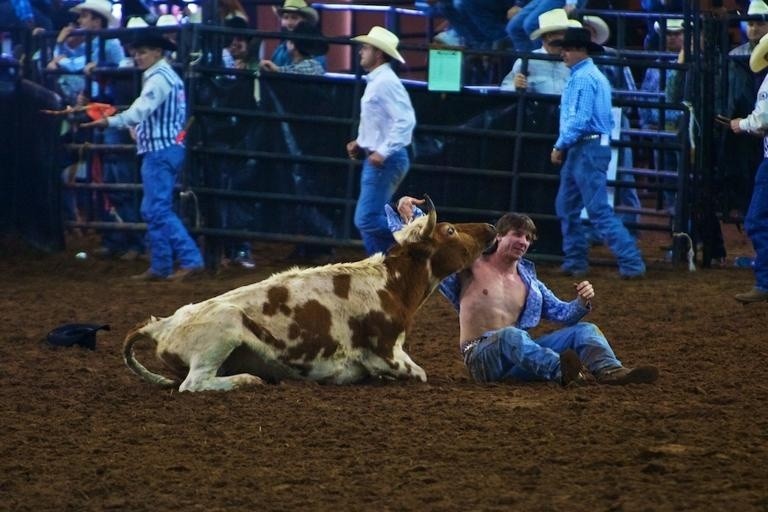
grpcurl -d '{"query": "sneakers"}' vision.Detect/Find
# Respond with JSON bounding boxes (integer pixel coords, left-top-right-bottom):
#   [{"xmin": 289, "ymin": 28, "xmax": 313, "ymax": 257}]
[
  {"xmin": 671, "ymin": 233, "xmax": 692, "ymax": 270},
  {"xmin": 732, "ymin": 287, "xmax": 767, "ymax": 304},
  {"xmin": 129, "ymin": 264, "xmax": 168, "ymax": 283},
  {"xmin": 228, "ymin": 242, "xmax": 259, "ymax": 268},
  {"xmin": 556, "ymin": 348, "xmax": 585, "ymax": 389},
  {"xmin": 734, "ymin": 256, "xmax": 757, "ymax": 269},
  {"xmin": 165, "ymin": 260, "xmax": 205, "ymax": 282},
  {"xmin": 593, "ymin": 363, "xmax": 660, "ymax": 386}
]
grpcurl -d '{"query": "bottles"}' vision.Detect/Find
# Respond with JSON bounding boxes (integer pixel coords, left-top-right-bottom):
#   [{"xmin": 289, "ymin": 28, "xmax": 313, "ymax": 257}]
[{"xmin": 724, "ymin": 253, "xmax": 755, "ymax": 270}]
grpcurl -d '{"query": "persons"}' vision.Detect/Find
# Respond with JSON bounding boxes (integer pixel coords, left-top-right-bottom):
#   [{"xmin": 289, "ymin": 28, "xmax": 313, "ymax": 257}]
[
  {"xmin": 551, "ymin": 29, "xmax": 645, "ymax": 277},
  {"xmin": 384, "ymin": 194, "xmax": 659, "ymax": 388},
  {"xmin": 348, "ymin": 27, "xmax": 416, "ymax": 255},
  {"xmin": 82, "ymin": 30, "xmax": 203, "ymax": 281},
  {"xmin": 0, "ymin": 2, "xmax": 330, "ymax": 260},
  {"xmin": 425, "ymin": 0, "xmax": 641, "ymax": 240},
  {"xmin": 637, "ymin": 0, "xmax": 768, "ymax": 304}
]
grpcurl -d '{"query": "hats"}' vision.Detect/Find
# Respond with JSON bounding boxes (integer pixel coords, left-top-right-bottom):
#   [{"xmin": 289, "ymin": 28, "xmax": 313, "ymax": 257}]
[
  {"xmin": 748, "ymin": 33, "xmax": 768, "ymax": 74},
  {"xmin": 277, "ymin": 0, "xmax": 320, "ymax": 25},
  {"xmin": 156, "ymin": 14, "xmax": 181, "ymax": 27},
  {"xmin": 546, "ymin": 27, "xmax": 605, "ymax": 55},
  {"xmin": 653, "ymin": 18, "xmax": 695, "ymax": 35},
  {"xmin": 735, "ymin": 0, "xmax": 768, "ymax": 19},
  {"xmin": 67, "ymin": 0, "xmax": 117, "ymax": 25},
  {"xmin": 527, "ymin": 7, "xmax": 583, "ymax": 41},
  {"xmin": 120, "ymin": 29, "xmax": 178, "ymax": 52},
  {"xmin": 45, "ymin": 323, "xmax": 112, "ymax": 352},
  {"xmin": 581, "ymin": 15, "xmax": 611, "ymax": 46},
  {"xmin": 126, "ymin": 16, "xmax": 157, "ymax": 29},
  {"xmin": 349, "ymin": 25, "xmax": 406, "ymax": 65}
]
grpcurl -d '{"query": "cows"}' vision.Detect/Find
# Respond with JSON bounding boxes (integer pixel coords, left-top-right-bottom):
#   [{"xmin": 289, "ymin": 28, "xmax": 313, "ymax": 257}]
[{"xmin": 120, "ymin": 192, "xmax": 499, "ymax": 395}]
